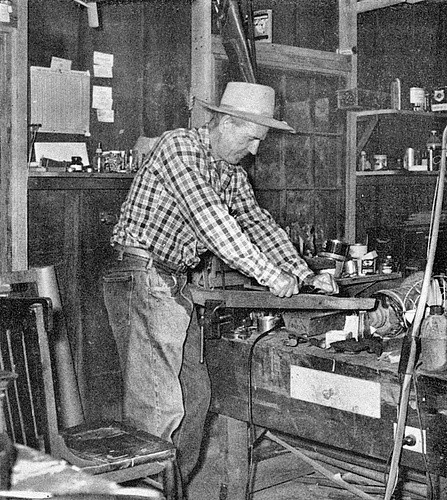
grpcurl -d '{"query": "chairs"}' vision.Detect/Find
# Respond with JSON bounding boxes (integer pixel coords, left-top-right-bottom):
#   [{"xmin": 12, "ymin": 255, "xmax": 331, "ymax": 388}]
[{"xmin": 0, "ymin": 292, "xmax": 184, "ymax": 500}]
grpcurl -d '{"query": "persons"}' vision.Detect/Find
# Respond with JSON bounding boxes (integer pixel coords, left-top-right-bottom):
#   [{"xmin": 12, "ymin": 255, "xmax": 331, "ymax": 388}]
[{"xmin": 102, "ymin": 80, "xmax": 339, "ymax": 484}]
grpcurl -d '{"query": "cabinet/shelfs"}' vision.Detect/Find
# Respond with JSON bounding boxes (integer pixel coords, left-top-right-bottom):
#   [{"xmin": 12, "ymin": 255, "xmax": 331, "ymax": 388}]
[{"xmin": 10, "ymin": 0, "xmax": 447, "ymax": 420}]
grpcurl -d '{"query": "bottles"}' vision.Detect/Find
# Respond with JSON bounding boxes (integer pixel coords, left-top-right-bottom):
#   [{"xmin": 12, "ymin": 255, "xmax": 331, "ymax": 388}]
[
  {"xmin": 358, "ymin": 151, "xmax": 367, "ymax": 170},
  {"xmin": 373, "ymin": 154, "xmax": 387, "ymax": 172},
  {"xmin": 93, "ymin": 149, "xmax": 105, "ymax": 173},
  {"xmin": 404, "ymin": 146, "xmax": 418, "ymax": 170},
  {"xmin": 427, "ymin": 130, "xmax": 441, "ymax": 171},
  {"xmin": 71, "ymin": 156, "xmax": 83, "ymax": 173},
  {"xmin": 421, "ymin": 306, "xmax": 447, "ymax": 373},
  {"xmin": 123, "ymin": 150, "xmax": 137, "ymax": 172}
]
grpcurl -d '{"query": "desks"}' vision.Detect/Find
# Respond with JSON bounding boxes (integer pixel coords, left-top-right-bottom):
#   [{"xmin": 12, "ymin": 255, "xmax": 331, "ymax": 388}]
[{"xmin": 218, "ymin": 332, "xmax": 447, "ymax": 500}]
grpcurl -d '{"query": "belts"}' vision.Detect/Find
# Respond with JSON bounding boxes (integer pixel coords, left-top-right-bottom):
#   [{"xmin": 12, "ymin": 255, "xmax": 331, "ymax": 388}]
[{"xmin": 113, "ymin": 243, "xmax": 177, "ymax": 273}]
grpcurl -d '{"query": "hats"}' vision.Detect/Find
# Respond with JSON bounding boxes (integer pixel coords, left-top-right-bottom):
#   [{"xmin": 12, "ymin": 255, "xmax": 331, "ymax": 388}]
[{"xmin": 193, "ymin": 82, "xmax": 297, "ymax": 133}]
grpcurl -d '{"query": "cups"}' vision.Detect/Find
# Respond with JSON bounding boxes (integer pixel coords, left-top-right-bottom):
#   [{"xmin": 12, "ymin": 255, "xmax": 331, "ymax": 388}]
[
  {"xmin": 325, "ymin": 239, "xmax": 367, "ymax": 276},
  {"xmin": 257, "ymin": 316, "xmax": 280, "ymax": 334}
]
